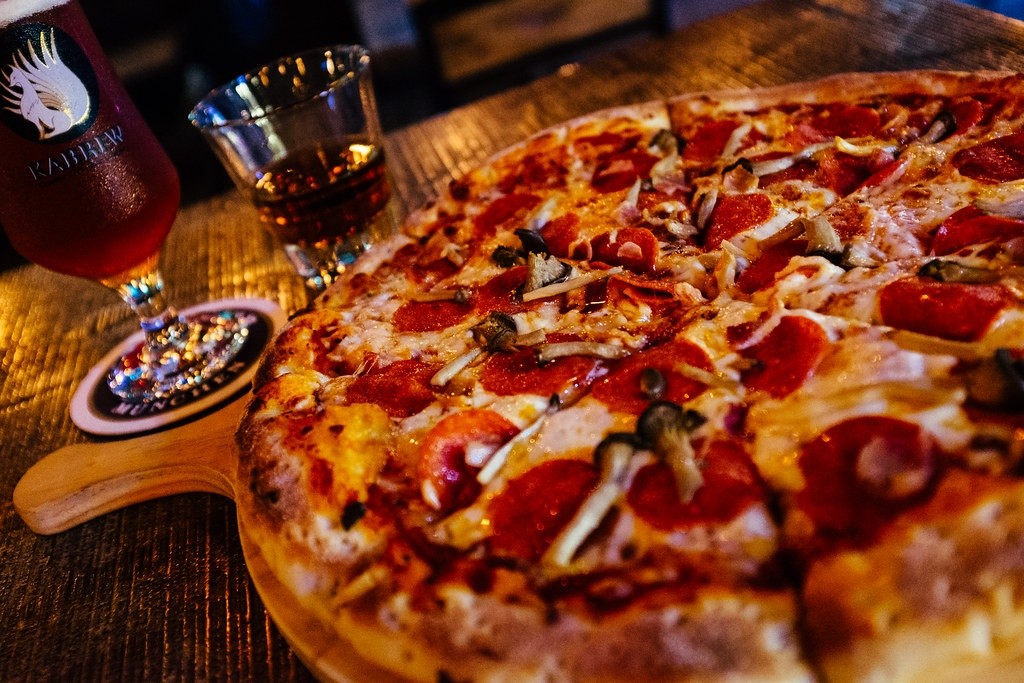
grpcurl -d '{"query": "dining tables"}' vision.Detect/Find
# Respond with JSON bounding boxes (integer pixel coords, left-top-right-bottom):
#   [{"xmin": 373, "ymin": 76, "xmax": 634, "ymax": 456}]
[{"xmin": 0, "ymin": 0, "xmax": 1024, "ymax": 683}]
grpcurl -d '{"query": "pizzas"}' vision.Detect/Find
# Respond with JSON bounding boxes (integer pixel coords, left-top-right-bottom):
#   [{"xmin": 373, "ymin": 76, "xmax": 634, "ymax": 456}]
[{"xmin": 232, "ymin": 70, "xmax": 1024, "ymax": 683}]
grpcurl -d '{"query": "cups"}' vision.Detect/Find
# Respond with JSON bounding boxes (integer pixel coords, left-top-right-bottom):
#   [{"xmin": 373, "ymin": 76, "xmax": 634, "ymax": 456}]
[{"xmin": 187, "ymin": 42, "xmax": 398, "ymax": 287}]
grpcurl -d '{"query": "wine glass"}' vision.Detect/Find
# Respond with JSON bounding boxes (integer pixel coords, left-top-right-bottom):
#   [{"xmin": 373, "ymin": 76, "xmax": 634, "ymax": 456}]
[{"xmin": 0, "ymin": 0, "xmax": 245, "ymax": 402}]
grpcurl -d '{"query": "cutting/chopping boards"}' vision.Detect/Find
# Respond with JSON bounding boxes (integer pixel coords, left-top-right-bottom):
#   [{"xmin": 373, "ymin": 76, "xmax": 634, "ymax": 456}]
[{"xmin": 13, "ymin": 390, "xmax": 409, "ymax": 683}]
